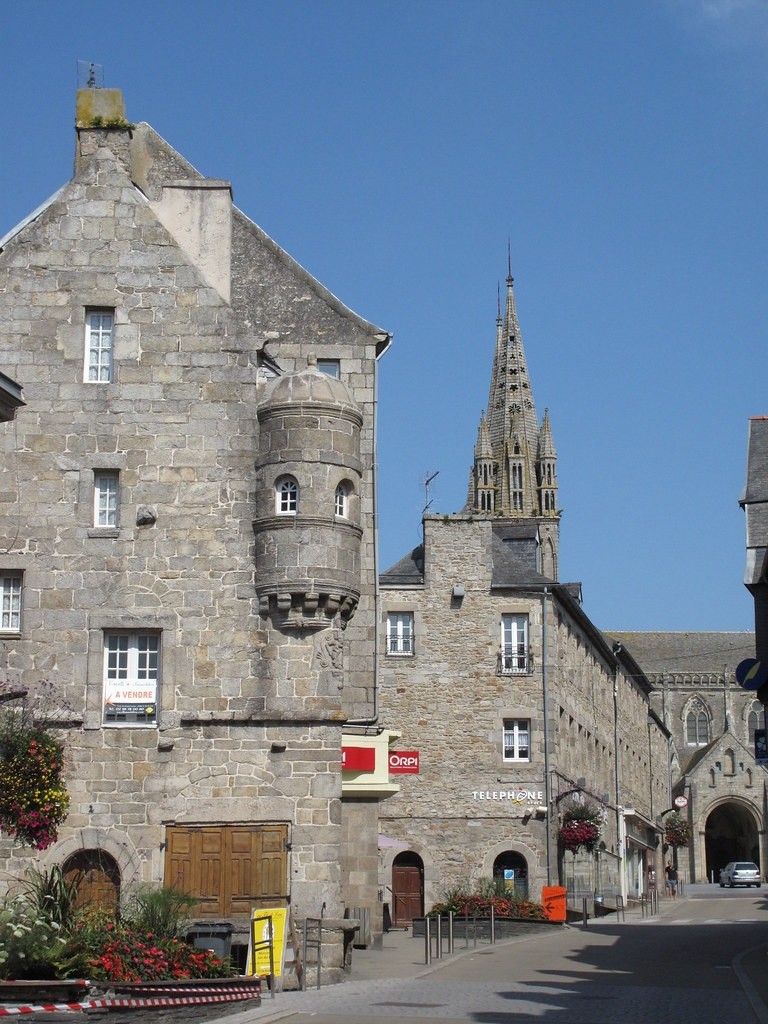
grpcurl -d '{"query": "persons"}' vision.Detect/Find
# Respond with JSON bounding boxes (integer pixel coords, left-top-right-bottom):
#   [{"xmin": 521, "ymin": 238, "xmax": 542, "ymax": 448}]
[{"xmin": 664, "ymin": 860, "xmax": 678, "ymax": 897}]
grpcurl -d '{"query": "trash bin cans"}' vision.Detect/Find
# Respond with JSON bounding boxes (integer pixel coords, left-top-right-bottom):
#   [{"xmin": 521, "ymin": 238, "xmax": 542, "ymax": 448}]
[{"xmin": 188, "ymin": 921, "xmax": 236, "ymax": 973}]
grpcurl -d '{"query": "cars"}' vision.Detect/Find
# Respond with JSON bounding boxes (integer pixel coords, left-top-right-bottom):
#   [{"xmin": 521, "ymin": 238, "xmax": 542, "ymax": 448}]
[
  {"xmin": 648, "ymin": 865, "xmax": 655, "ymax": 884},
  {"xmin": 719, "ymin": 862, "xmax": 760, "ymax": 888}
]
